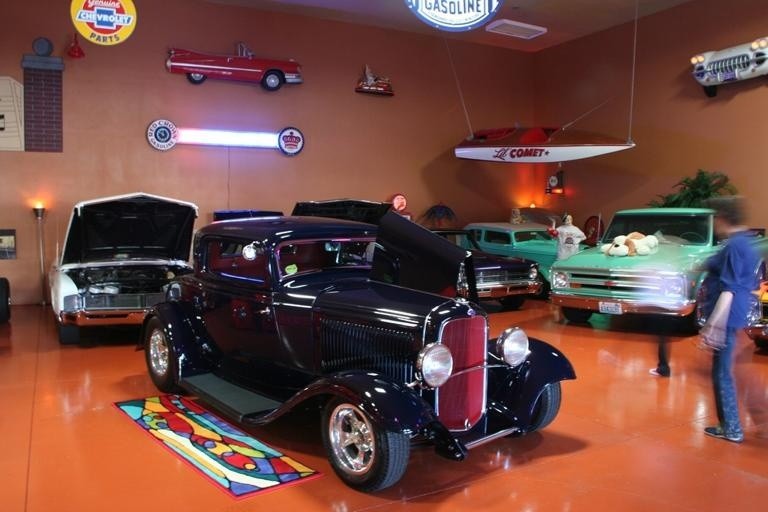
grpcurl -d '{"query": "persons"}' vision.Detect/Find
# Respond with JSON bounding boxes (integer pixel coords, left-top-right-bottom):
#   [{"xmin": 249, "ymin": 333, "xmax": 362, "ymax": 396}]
[
  {"xmin": 689, "ymin": 196, "xmax": 762, "ymax": 443},
  {"xmin": 546, "ymin": 215, "xmax": 587, "ymax": 263}
]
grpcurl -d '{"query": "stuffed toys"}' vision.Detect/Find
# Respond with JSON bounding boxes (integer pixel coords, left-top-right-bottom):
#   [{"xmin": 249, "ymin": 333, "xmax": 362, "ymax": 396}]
[{"xmin": 600, "ymin": 231, "xmax": 659, "ymax": 257}]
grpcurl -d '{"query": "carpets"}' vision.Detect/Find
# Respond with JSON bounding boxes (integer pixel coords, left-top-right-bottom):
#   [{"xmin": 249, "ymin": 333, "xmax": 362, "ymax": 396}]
[{"xmin": 112, "ymin": 393, "xmax": 326, "ymax": 501}]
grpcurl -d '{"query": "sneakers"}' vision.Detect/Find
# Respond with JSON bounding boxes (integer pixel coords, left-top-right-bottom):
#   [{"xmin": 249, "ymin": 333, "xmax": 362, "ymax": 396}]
[
  {"xmin": 704, "ymin": 427, "xmax": 743, "ymax": 442},
  {"xmin": 649, "ymin": 369, "xmax": 661, "ymax": 375}
]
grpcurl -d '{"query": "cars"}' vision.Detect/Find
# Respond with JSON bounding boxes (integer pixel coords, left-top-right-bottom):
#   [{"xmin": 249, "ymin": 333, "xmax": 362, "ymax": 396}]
[
  {"xmin": 427, "ymin": 227, "xmax": 545, "ymax": 311},
  {"xmin": 462, "ymin": 221, "xmax": 593, "ymax": 299},
  {"xmin": 48, "ymin": 191, "xmax": 200, "ymax": 346},
  {"xmin": 547, "ymin": 207, "xmax": 768, "ymax": 335}
]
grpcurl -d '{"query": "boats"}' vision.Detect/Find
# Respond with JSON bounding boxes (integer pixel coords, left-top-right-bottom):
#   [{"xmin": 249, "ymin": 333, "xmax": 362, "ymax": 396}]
[{"xmin": 454, "ymin": 126, "xmax": 637, "ymax": 164}]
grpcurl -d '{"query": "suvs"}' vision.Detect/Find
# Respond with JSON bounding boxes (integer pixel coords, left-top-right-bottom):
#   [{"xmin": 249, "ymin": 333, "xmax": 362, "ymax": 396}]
[{"xmin": 139, "ymin": 215, "xmax": 577, "ymax": 494}]
